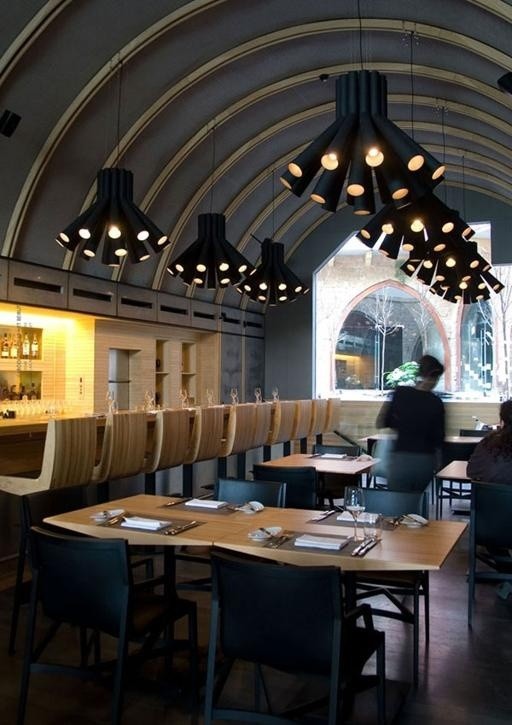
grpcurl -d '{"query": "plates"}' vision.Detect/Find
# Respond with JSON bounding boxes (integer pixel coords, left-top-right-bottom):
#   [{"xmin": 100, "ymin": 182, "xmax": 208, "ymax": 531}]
[
  {"xmin": 248, "ymin": 527, "xmax": 282, "ymax": 542},
  {"xmin": 235, "ymin": 501, "xmax": 264, "ymax": 513},
  {"xmin": 89, "ymin": 509, "xmax": 125, "ymax": 522}
]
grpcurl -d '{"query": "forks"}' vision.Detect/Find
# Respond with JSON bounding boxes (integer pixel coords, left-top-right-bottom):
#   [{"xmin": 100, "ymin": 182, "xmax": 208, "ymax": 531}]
[{"xmin": 258, "ymin": 527, "xmax": 295, "ymax": 548}]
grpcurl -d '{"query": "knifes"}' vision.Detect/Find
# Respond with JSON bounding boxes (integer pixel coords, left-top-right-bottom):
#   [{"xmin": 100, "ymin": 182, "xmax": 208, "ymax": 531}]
[
  {"xmin": 96, "ymin": 511, "xmax": 129, "ymax": 526},
  {"xmin": 166, "ymin": 497, "xmax": 192, "ymax": 505},
  {"xmin": 352, "ymin": 536, "xmax": 380, "ymax": 556},
  {"xmin": 245, "ymin": 501, "xmax": 255, "ymax": 512},
  {"xmin": 164, "ymin": 520, "xmax": 208, "ymax": 535},
  {"xmin": 312, "ymin": 510, "xmax": 335, "ymax": 521}
]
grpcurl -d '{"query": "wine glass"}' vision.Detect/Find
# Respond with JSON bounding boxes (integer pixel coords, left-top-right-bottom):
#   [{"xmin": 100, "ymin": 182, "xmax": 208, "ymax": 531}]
[
  {"xmin": 344, "ymin": 487, "xmax": 365, "ymax": 542},
  {"xmin": 157, "ymin": 358, "xmax": 160, "ymax": 371},
  {"xmin": 143, "ymin": 389, "xmax": 161, "ymax": 413},
  {"xmin": 105, "ymin": 391, "xmax": 118, "ymax": 415},
  {"xmin": 231, "ymin": 387, "xmax": 279, "ymax": 405},
  {"xmin": 177, "ymin": 388, "xmax": 189, "ymax": 411},
  {"xmin": 204, "ymin": 388, "xmax": 217, "ymax": 408}
]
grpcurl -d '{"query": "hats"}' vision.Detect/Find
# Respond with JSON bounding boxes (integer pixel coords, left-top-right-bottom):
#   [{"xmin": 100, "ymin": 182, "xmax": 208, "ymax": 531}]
[{"xmin": 415, "ymin": 354, "xmax": 445, "ymax": 378}]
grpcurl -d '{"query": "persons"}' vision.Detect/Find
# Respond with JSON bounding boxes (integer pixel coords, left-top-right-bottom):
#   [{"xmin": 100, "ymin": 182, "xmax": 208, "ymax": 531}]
[
  {"xmin": 377, "ymin": 355, "xmax": 446, "ymax": 503},
  {"xmin": 467, "ymin": 400, "xmax": 512, "ymax": 598}
]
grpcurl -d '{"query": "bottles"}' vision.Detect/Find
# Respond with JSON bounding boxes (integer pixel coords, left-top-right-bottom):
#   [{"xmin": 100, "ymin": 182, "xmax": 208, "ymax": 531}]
[
  {"xmin": 1, "ymin": 332, "xmax": 39, "ymax": 360},
  {"xmin": 0, "ymin": 380, "xmax": 72, "ymax": 420}
]
